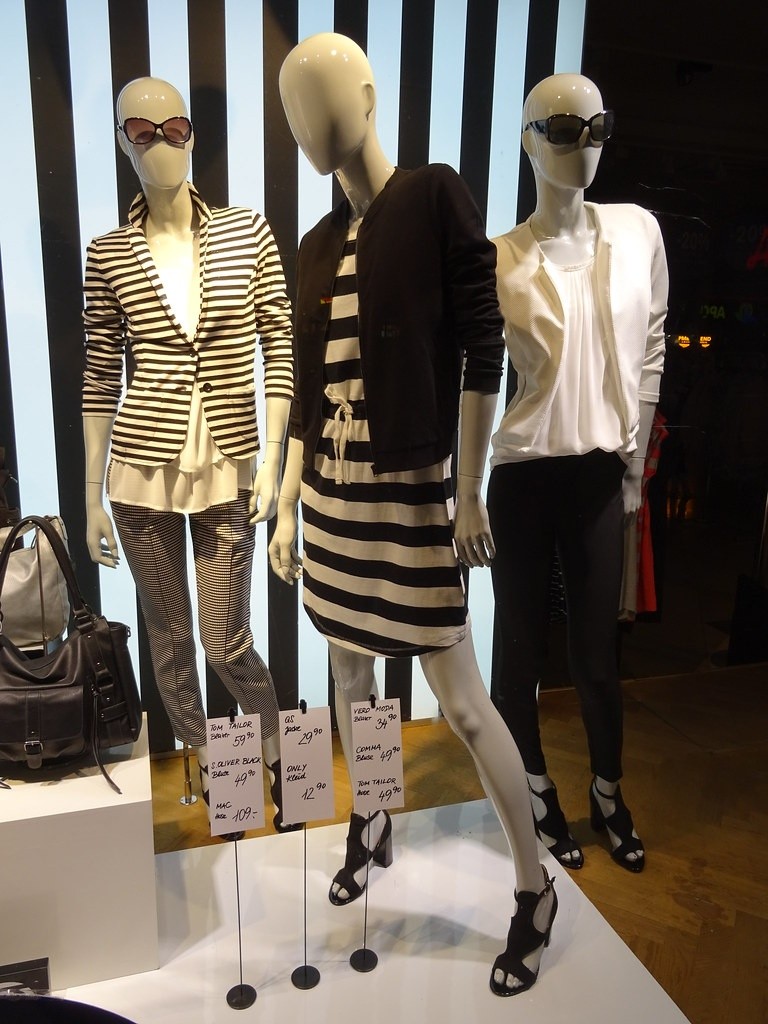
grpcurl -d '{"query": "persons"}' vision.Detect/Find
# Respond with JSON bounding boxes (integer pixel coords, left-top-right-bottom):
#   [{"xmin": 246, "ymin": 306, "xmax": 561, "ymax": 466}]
[
  {"xmin": 80, "ymin": 75, "xmax": 304, "ymax": 841},
  {"xmin": 486, "ymin": 73, "xmax": 670, "ymax": 873},
  {"xmin": 267, "ymin": 32, "xmax": 558, "ymax": 998}
]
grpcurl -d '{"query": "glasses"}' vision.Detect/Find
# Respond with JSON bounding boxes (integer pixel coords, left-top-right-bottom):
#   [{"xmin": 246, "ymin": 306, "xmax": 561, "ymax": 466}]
[
  {"xmin": 118, "ymin": 117, "xmax": 193, "ymax": 146},
  {"xmin": 525, "ymin": 108, "xmax": 616, "ymax": 144}
]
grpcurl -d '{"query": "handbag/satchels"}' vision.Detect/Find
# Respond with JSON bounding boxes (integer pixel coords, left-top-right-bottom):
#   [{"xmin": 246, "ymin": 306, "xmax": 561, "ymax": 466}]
[{"xmin": 0, "ymin": 514, "xmax": 142, "ymax": 795}]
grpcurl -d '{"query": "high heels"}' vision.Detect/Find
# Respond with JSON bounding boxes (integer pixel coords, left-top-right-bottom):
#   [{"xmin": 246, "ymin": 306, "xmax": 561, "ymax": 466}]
[
  {"xmin": 590, "ymin": 782, "xmax": 646, "ymax": 875},
  {"xmin": 329, "ymin": 806, "xmax": 394, "ymax": 906},
  {"xmin": 263, "ymin": 760, "xmax": 304, "ymax": 834},
  {"xmin": 489, "ymin": 865, "xmax": 559, "ymax": 997},
  {"xmin": 199, "ymin": 764, "xmax": 246, "ymax": 841},
  {"xmin": 528, "ymin": 778, "xmax": 583, "ymax": 869}
]
